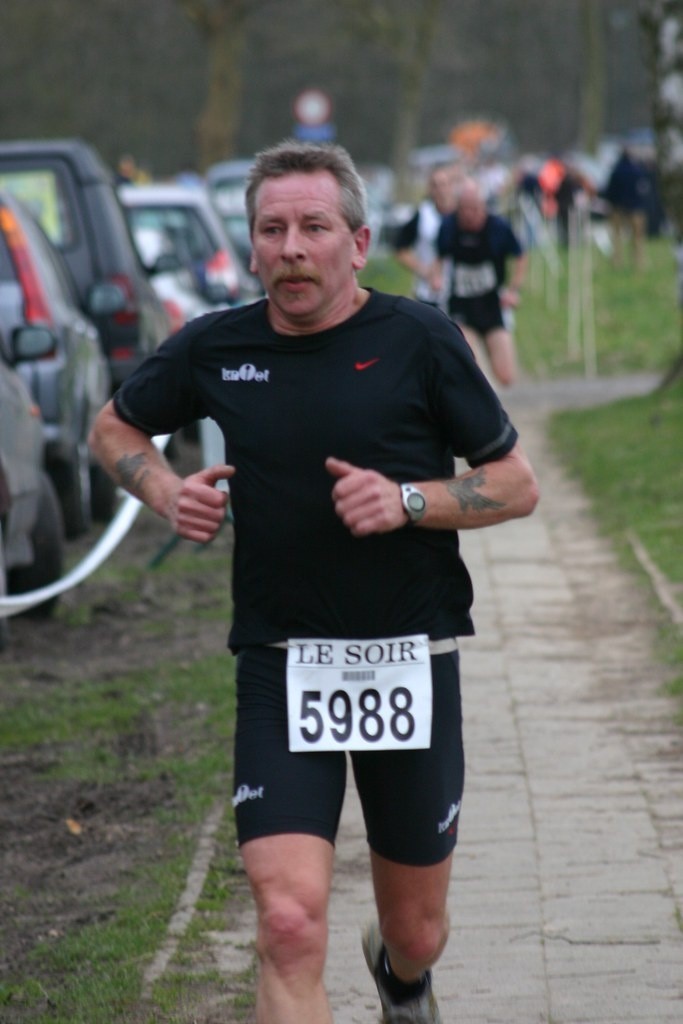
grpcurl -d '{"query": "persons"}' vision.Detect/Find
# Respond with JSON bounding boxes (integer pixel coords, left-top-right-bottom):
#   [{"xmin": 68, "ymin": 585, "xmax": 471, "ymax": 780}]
[
  {"xmin": 388, "ymin": 134, "xmax": 665, "ymax": 389},
  {"xmin": 87, "ymin": 144, "xmax": 541, "ymax": 1024}
]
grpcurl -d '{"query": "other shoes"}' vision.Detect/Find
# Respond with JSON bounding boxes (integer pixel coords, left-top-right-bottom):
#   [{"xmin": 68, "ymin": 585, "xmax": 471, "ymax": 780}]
[{"xmin": 360, "ymin": 915, "xmax": 443, "ymax": 1024}]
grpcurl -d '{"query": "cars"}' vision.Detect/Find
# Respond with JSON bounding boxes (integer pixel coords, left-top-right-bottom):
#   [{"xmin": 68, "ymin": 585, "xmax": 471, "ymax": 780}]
[{"xmin": 1, "ymin": 129, "xmax": 672, "ymax": 627}]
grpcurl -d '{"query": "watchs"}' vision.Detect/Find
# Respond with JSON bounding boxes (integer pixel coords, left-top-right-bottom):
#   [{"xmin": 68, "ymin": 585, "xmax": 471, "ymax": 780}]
[{"xmin": 398, "ymin": 480, "xmax": 428, "ymax": 523}]
